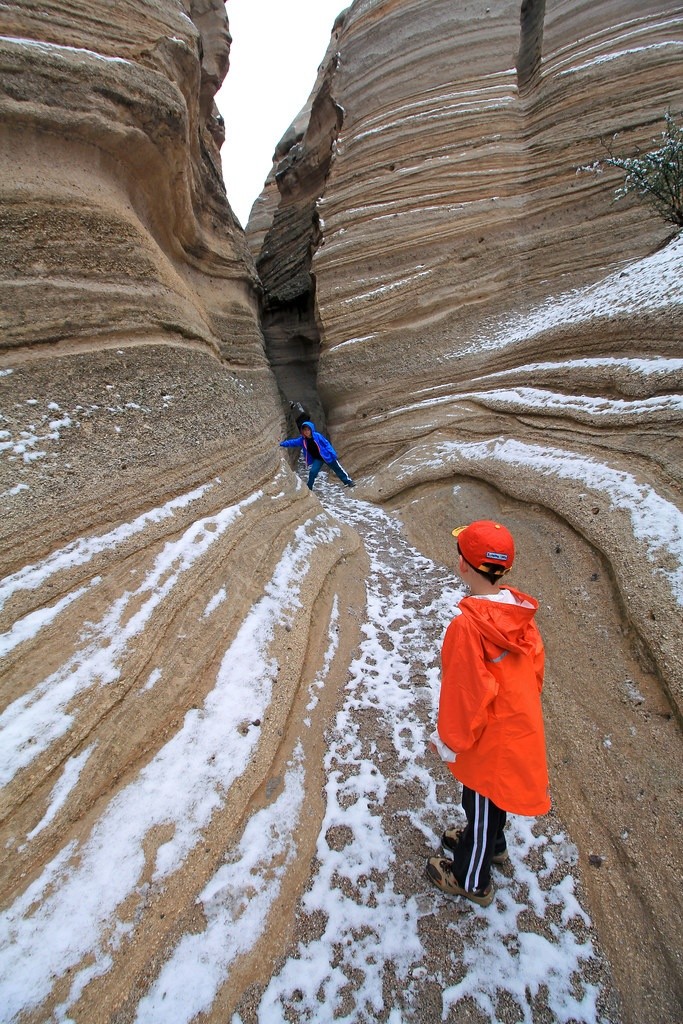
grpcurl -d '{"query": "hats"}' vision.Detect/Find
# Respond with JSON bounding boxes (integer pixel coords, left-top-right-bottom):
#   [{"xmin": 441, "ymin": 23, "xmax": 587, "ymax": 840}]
[{"xmin": 451, "ymin": 520, "xmax": 515, "ymax": 574}]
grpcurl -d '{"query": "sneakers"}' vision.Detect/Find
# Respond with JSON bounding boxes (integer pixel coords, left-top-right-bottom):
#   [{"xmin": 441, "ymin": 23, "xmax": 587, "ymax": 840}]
[
  {"xmin": 442, "ymin": 828, "xmax": 508, "ymax": 863},
  {"xmin": 426, "ymin": 857, "xmax": 495, "ymax": 906},
  {"xmin": 348, "ymin": 481, "xmax": 356, "ymax": 487}
]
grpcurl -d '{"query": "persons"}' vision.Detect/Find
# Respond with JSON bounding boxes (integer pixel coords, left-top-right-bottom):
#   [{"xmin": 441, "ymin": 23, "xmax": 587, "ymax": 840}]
[
  {"xmin": 425, "ymin": 519, "xmax": 552, "ymax": 911},
  {"xmin": 271, "ymin": 421, "xmax": 356, "ymax": 490},
  {"xmin": 289, "ymin": 401, "xmax": 310, "ymax": 433}
]
